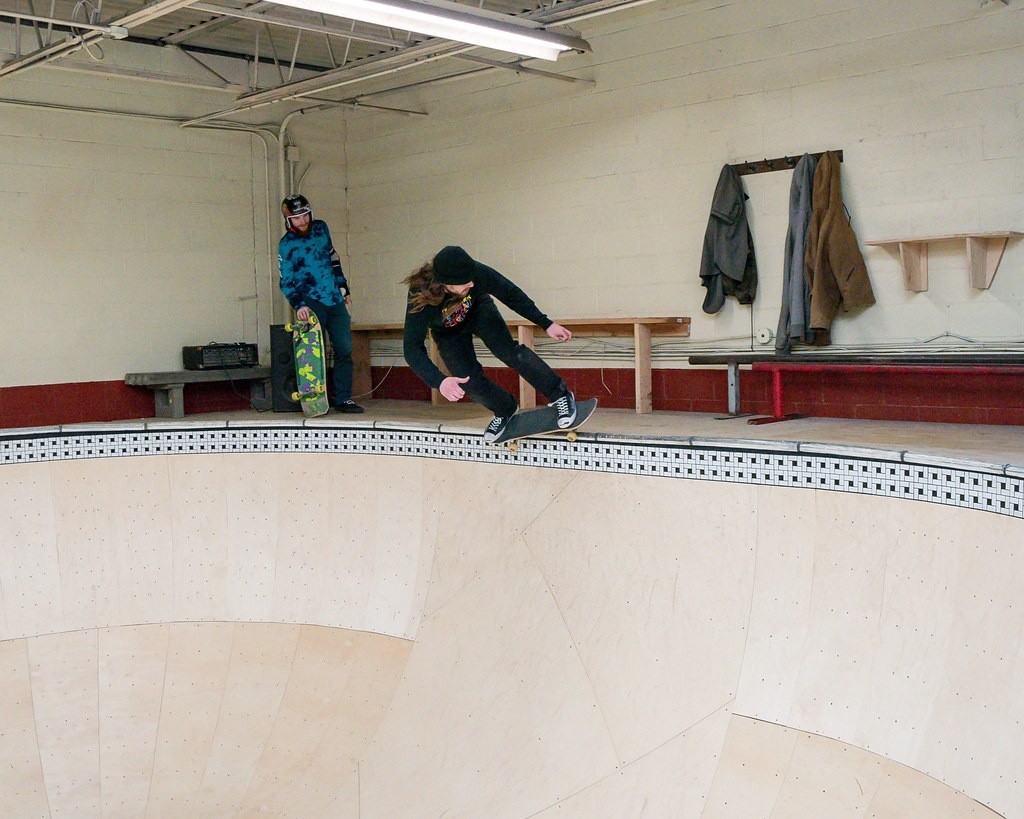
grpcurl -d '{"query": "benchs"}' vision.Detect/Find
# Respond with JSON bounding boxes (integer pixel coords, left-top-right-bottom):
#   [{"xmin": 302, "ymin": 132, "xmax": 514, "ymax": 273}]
[{"xmin": 124, "ymin": 365, "xmax": 271, "ymax": 419}]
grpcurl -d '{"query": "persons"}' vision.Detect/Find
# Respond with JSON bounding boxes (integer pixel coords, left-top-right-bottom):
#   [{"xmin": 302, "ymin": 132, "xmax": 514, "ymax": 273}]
[
  {"xmin": 393, "ymin": 245, "xmax": 577, "ymax": 443},
  {"xmin": 277, "ymin": 194, "xmax": 366, "ymax": 413}
]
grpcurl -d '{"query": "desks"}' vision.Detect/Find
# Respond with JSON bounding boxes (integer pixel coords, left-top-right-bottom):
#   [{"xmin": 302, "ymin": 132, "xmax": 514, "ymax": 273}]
[{"xmin": 350, "ymin": 317, "xmax": 690, "ymax": 414}]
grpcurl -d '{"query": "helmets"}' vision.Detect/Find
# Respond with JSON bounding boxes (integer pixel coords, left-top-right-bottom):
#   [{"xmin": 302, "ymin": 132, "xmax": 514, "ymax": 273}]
[{"xmin": 280, "ymin": 195, "xmax": 315, "ymax": 228}]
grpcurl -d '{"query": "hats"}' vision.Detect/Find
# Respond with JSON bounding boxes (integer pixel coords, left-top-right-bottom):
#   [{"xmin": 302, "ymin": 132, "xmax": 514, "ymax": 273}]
[{"xmin": 434, "ymin": 247, "xmax": 475, "ymax": 286}]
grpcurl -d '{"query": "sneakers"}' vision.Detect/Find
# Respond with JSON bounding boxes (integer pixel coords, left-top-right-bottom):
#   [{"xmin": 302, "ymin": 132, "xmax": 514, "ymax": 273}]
[
  {"xmin": 334, "ymin": 402, "xmax": 365, "ymax": 413},
  {"xmin": 556, "ymin": 390, "xmax": 577, "ymax": 428},
  {"xmin": 483, "ymin": 404, "xmax": 521, "ymax": 442}
]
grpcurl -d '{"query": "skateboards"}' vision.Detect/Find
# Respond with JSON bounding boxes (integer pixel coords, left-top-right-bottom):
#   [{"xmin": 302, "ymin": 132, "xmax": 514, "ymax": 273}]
[
  {"xmin": 285, "ymin": 310, "xmax": 329, "ymax": 417},
  {"xmin": 493, "ymin": 396, "xmax": 599, "ymax": 452}
]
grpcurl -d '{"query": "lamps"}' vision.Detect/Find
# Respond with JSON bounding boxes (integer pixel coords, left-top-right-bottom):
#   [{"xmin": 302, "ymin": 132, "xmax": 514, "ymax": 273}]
[{"xmin": 260, "ymin": 0, "xmax": 594, "ymax": 62}]
